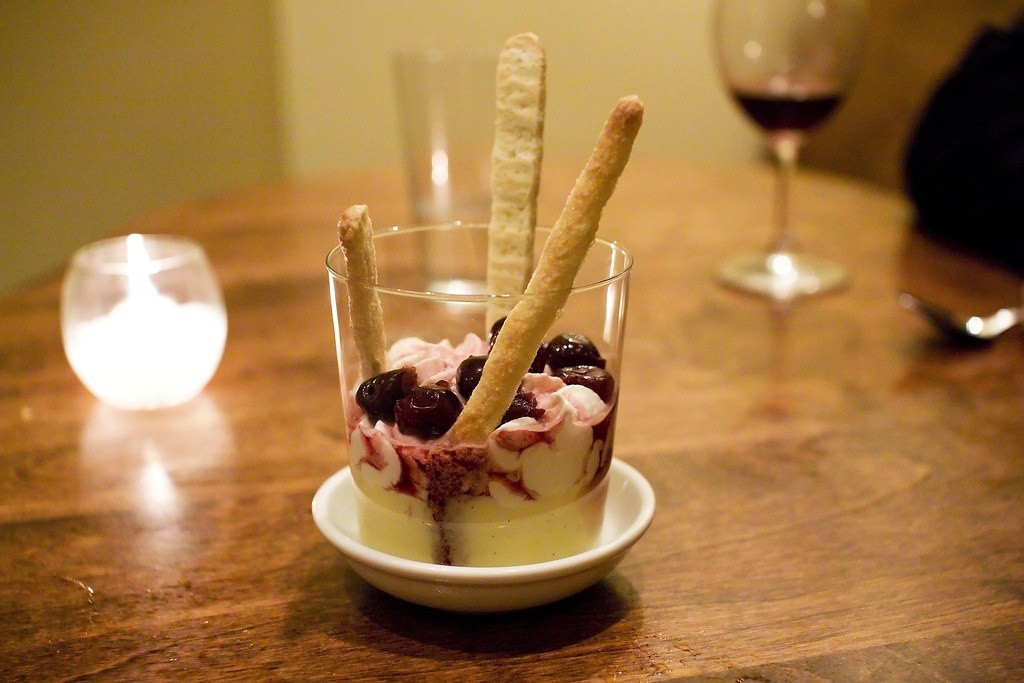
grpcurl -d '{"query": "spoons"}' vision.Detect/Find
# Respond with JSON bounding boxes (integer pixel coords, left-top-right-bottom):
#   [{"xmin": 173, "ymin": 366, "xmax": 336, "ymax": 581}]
[{"xmin": 902, "ymin": 291, "xmax": 1024, "ymax": 342}]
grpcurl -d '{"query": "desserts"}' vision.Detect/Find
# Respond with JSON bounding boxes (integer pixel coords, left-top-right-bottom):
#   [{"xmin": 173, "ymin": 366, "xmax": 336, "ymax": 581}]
[{"xmin": 325, "ymin": 31, "xmax": 645, "ymax": 567}]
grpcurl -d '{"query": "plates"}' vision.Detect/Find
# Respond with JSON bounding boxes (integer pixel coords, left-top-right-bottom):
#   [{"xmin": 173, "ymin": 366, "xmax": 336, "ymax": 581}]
[{"xmin": 310, "ymin": 454, "xmax": 655, "ymax": 610}]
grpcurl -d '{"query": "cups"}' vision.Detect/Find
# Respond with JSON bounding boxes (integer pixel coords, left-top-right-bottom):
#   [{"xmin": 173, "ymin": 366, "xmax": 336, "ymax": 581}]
[
  {"xmin": 60, "ymin": 234, "xmax": 229, "ymax": 409},
  {"xmin": 325, "ymin": 225, "xmax": 634, "ymax": 566},
  {"xmin": 397, "ymin": 54, "xmax": 498, "ymax": 311}
]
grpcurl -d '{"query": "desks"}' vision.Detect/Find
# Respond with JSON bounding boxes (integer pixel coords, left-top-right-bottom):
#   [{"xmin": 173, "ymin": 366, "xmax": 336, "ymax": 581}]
[{"xmin": 0, "ymin": 155, "xmax": 1024, "ymax": 683}]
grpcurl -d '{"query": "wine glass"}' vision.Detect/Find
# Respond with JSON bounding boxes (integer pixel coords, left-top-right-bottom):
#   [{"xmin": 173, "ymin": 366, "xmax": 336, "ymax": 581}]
[{"xmin": 712, "ymin": 0, "xmax": 868, "ymax": 298}]
